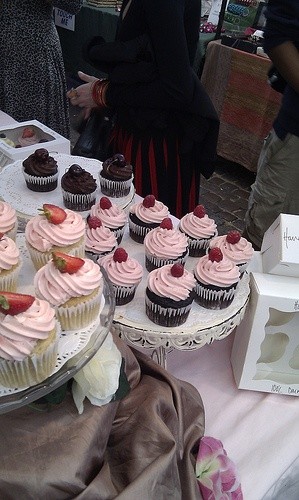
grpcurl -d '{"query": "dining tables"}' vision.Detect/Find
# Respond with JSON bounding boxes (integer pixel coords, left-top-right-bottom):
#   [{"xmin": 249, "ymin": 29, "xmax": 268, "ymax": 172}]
[{"xmin": 0, "ymin": 112, "xmax": 299, "ymax": 500}]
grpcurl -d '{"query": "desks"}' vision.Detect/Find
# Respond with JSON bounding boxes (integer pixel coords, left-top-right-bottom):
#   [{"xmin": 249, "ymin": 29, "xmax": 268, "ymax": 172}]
[{"xmin": 201, "ymin": 40, "xmax": 283, "ymax": 174}]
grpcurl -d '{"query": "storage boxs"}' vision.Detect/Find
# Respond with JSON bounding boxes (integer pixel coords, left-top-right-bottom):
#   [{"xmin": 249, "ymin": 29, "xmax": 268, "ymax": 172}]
[
  {"xmin": 0, "ymin": 119, "xmax": 70, "ymax": 160},
  {"xmin": 229, "ymin": 213, "xmax": 299, "ymax": 397}
]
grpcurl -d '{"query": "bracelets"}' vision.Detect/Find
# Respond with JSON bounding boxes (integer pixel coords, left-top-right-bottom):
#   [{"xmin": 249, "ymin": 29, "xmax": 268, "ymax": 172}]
[{"xmin": 91, "ymin": 78, "xmax": 108, "ymax": 108}]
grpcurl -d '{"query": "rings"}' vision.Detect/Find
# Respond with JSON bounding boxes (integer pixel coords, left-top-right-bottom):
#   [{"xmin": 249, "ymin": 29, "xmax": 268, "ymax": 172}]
[{"xmin": 70, "ymin": 87, "xmax": 80, "ymax": 98}]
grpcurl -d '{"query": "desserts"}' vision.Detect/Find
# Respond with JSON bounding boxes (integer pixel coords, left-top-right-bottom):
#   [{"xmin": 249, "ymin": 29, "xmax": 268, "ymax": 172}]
[
  {"xmin": 82, "ymin": 195, "xmax": 255, "ymax": 329},
  {"xmin": 22, "ymin": 148, "xmax": 58, "ymax": 192},
  {"xmin": 100, "ymin": 153, "xmax": 138, "ymax": 198},
  {"xmin": 61, "ymin": 164, "xmax": 98, "ymax": 212},
  {"xmin": 0, "ymin": 198, "xmax": 103, "ymax": 389}
]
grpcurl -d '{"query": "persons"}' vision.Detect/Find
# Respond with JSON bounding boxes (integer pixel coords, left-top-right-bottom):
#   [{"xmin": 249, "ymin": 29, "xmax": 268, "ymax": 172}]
[
  {"xmin": 243, "ymin": 0, "xmax": 299, "ymax": 251},
  {"xmin": 67, "ymin": 0, "xmax": 218, "ymax": 217},
  {"xmin": 0, "ymin": 0, "xmax": 83, "ymax": 137}
]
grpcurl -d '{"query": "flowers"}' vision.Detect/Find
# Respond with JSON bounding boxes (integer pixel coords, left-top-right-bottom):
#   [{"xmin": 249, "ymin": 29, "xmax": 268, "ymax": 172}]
[
  {"xmin": 71, "ymin": 331, "xmax": 123, "ymax": 414},
  {"xmin": 195, "ymin": 437, "xmax": 244, "ymax": 500}
]
